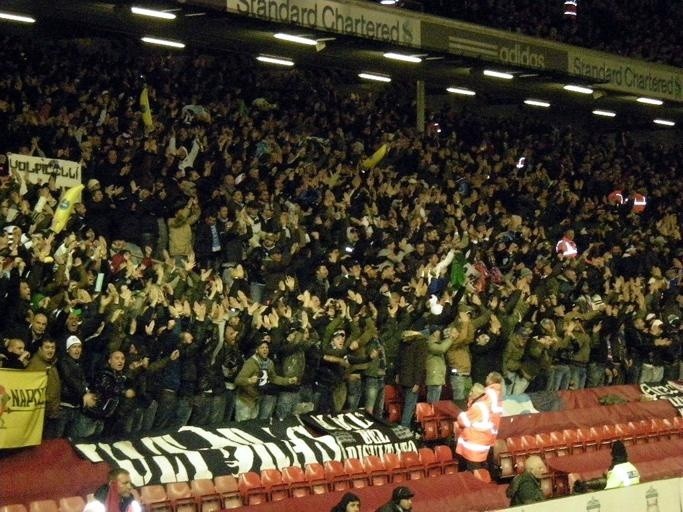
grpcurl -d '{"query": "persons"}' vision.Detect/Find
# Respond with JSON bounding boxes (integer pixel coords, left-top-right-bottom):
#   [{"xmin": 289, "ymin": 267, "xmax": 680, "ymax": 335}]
[
  {"xmin": 331, "ymin": 492, "xmax": 360, "ymax": 512},
  {"xmin": 453, "ymin": 372, "xmax": 504, "ymax": 472},
  {"xmin": 0, "ymin": 1, "xmax": 682, "ymax": 437},
  {"xmin": 603, "ymin": 441, "xmax": 640, "ymax": 489},
  {"xmin": 507, "ymin": 455, "xmax": 546, "ymax": 507},
  {"xmin": 375, "ymin": 486, "xmax": 416, "ymax": 512},
  {"xmin": 83, "ymin": 467, "xmax": 145, "ymax": 512}
]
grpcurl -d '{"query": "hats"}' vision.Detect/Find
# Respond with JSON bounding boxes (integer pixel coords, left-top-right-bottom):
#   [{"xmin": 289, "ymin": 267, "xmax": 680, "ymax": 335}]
[
  {"xmin": 392, "ymin": 485, "xmax": 415, "ymax": 505},
  {"xmin": 610, "ymin": 440, "xmax": 628, "ymax": 459},
  {"xmin": 65, "ymin": 335, "xmax": 82, "ymax": 350}
]
